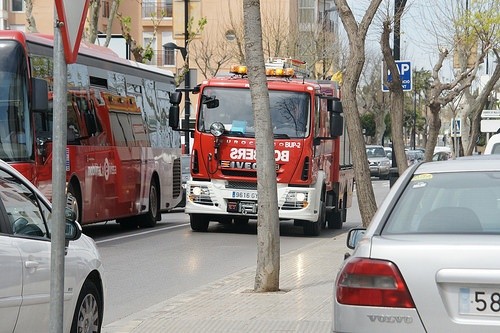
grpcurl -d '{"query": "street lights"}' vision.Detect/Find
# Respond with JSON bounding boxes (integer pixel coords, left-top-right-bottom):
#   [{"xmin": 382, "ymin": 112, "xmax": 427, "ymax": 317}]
[{"xmin": 412, "ymin": 65, "xmax": 423, "ymax": 150}]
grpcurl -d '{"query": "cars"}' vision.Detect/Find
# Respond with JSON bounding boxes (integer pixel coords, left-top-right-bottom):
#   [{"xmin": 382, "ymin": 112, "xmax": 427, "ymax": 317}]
[
  {"xmin": 0, "ymin": 159, "xmax": 107, "ymax": 333},
  {"xmin": 332, "ymin": 152, "xmax": 500, "ymax": 333},
  {"xmin": 399, "ymin": 147, "xmax": 454, "ymax": 169}
]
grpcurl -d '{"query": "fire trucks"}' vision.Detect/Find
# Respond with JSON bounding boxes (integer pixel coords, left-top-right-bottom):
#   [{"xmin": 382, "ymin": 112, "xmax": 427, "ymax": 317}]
[{"xmin": 182, "ymin": 56, "xmax": 355, "ymax": 236}]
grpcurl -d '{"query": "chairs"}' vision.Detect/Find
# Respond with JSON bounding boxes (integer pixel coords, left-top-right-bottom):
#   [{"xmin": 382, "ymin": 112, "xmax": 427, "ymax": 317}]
[{"xmin": 415, "ymin": 205, "xmax": 484, "ymax": 234}]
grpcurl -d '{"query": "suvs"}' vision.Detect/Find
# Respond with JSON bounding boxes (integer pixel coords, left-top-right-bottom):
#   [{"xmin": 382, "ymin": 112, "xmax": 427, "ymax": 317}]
[{"xmin": 361, "ymin": 144, "xmax": 391, "ymax": 180}]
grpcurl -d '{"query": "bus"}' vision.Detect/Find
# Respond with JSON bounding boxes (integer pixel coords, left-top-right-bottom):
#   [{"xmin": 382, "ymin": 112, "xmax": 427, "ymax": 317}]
[{"xmin": 0, "ymin": 27, "xmax": 184, "ymax": 229}]
